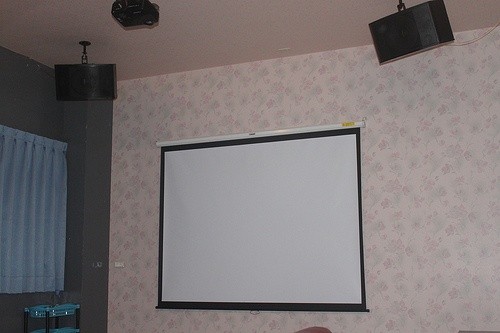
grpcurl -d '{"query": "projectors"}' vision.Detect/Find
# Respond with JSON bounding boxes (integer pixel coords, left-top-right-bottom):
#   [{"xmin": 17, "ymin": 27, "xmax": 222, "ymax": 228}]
[{"xmin": 112, "ymin": 1, "xmax": 160, "ymax": 28}]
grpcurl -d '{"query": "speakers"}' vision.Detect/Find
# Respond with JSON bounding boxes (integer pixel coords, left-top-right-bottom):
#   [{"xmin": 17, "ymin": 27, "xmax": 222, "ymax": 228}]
[
  {"xmin": 369, "ymin": 0, "xmax": 454, "ymax": 66},
  {"xmin": 53, "ymin": 64, "xmax": 118, "ymax": 102}
]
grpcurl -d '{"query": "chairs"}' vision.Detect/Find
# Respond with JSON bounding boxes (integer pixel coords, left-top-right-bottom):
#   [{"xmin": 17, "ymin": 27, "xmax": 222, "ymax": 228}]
[
  {"xmin": 24, "ymin": 304, "xmax": 60, "ymax": 332},
  {"xmin": 46, "ymin": 303, "xmax": 81, "ymax": 332}
]
